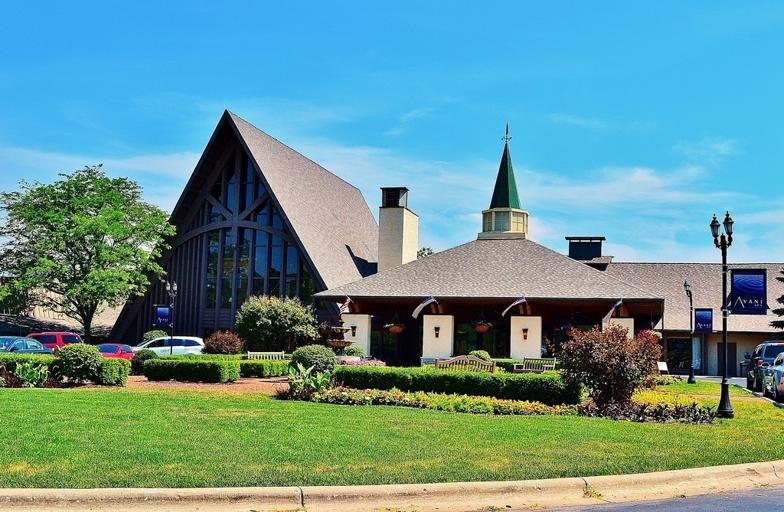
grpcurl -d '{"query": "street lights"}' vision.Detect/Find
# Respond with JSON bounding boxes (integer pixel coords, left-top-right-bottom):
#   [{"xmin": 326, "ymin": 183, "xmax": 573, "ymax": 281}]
[
  {"xmin": 710, "ymin": 210, "xmax": 735, "ymax": 419},
  {"xmin": 165, "ymin": 279, "xmax": 178, "ymax": 356},
  {"xmin": 683, "ymin": 280, "xmax": 696, "ymax": 384}
]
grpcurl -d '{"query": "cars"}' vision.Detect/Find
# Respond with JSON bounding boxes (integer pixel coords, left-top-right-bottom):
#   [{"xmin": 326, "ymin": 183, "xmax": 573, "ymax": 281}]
[
  {"xmin": 0, "ymin": 331, "xmax": 205, "ymax": 360},
  {"xmin": 743, "ymin": 340, "xmax": 784, "ymax": 400}
]
